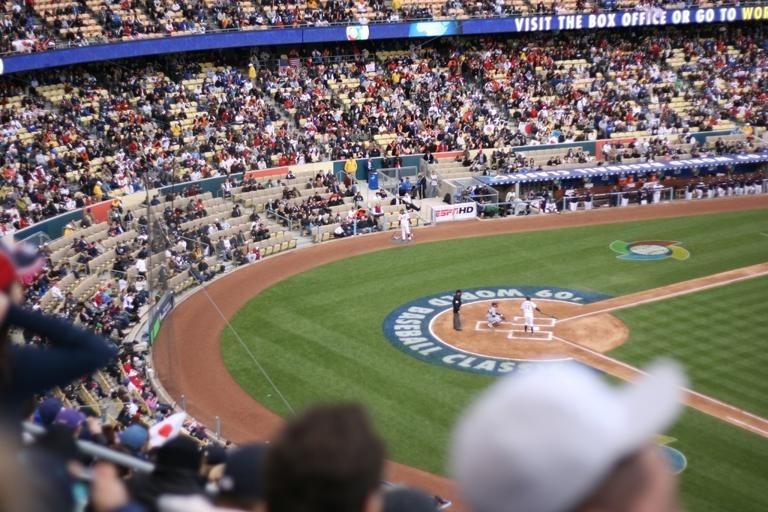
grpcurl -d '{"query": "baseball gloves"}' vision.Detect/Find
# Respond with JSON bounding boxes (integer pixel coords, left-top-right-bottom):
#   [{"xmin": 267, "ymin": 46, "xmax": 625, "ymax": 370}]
[{"xmin": 500, "ymin": 316, "xmax": 505, "ymax": 320}]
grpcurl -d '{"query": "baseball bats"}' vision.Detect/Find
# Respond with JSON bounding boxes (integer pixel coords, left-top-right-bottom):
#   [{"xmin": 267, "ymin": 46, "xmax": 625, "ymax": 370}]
[{"xmin": 540, "ymin": 312, "xmax": 558, "ymax": 320}]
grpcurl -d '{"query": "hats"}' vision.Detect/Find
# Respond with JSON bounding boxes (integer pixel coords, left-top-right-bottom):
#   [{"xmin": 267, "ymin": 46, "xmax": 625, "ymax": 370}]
[
  {"xmin": 0, "ymin": 235, "xmax": 43, "ymax": 291},
  {"xmin": 448, "ymin": 356, "xmax": 688, "ymax": 512}
]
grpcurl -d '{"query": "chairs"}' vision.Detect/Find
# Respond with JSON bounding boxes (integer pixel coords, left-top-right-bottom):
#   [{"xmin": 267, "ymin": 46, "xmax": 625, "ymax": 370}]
[
  {"xmin": 4, "ymin": 37, "xmax": 467, "ymax": 261},
  {"xmin": 1, "ymin": 3, "xmax": 466, "ymax": 54},
  {"xmin": 17, "ymin": 262, "xmax": 225, "ymax": 318}
]
